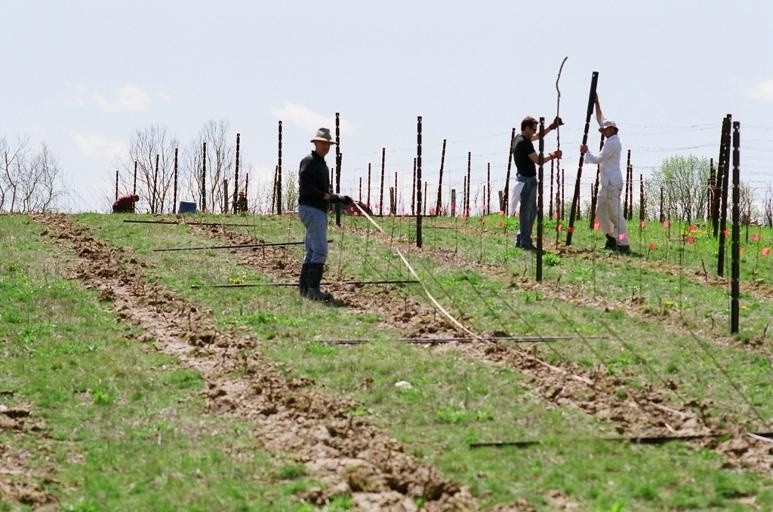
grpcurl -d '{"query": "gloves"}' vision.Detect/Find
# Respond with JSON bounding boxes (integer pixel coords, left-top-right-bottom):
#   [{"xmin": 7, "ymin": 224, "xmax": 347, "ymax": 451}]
[
  {"xmin": 548, "ymin": 116, "xmax": 564, "ymax": 131},
  {"xmin": 328, "ymin": 192, "xmax": 354, "ymax": 206}
]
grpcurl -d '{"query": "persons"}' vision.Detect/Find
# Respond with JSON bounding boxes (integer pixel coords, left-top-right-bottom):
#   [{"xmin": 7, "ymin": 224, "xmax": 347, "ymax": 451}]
[
  {"xmin": 579, "ymin": 90, "xmax": 629, "ymax": 253},
  {"xmin": 509, "ymin": 116, "xmax": 565, "ymax": 250},
  {"xmin": 112, "ymin": 191, "xmax": 139, "ymax": 214},
  {"xmin": 294, "ymin": 126, "xmax": 352, "ymax": 302},
  {"xmin": 236, "ymin": 191, "xmax": 248, "ymax": 214}
]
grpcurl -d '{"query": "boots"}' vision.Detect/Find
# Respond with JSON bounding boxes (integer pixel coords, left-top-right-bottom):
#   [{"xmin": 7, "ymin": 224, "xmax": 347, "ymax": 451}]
[
  {"xmin": 305, "ymin": 262, "xmax": 330, "ymax": 301},
  {"xmin": 298, "ymin": 261, "xmax": 309, "ymax": 299}
]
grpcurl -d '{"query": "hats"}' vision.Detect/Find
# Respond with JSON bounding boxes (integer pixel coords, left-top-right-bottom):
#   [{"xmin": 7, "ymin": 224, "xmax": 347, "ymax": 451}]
[
  {"xmin": 309, "ymin": 126, "xmax": 339, "ymax": 146},
  {"xmin": 597, "ymin": 119, "xmax": 618, "ymax": 134}
]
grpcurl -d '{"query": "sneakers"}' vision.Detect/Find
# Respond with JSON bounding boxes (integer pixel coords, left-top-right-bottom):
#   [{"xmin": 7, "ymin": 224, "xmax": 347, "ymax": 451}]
[
  {"xmin": 514, "ymin": 235, "xmax": 547, "ymax": 255},
  {"xmin": 604, "ymin": 233, "xmax": 631, "ymax": 252}
]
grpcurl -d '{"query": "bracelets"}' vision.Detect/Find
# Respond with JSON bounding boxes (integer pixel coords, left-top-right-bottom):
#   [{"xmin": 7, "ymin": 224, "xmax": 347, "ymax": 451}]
[{"xmin": 548, "ymin": 153, "xmax": 553, "ymax": 160}]
